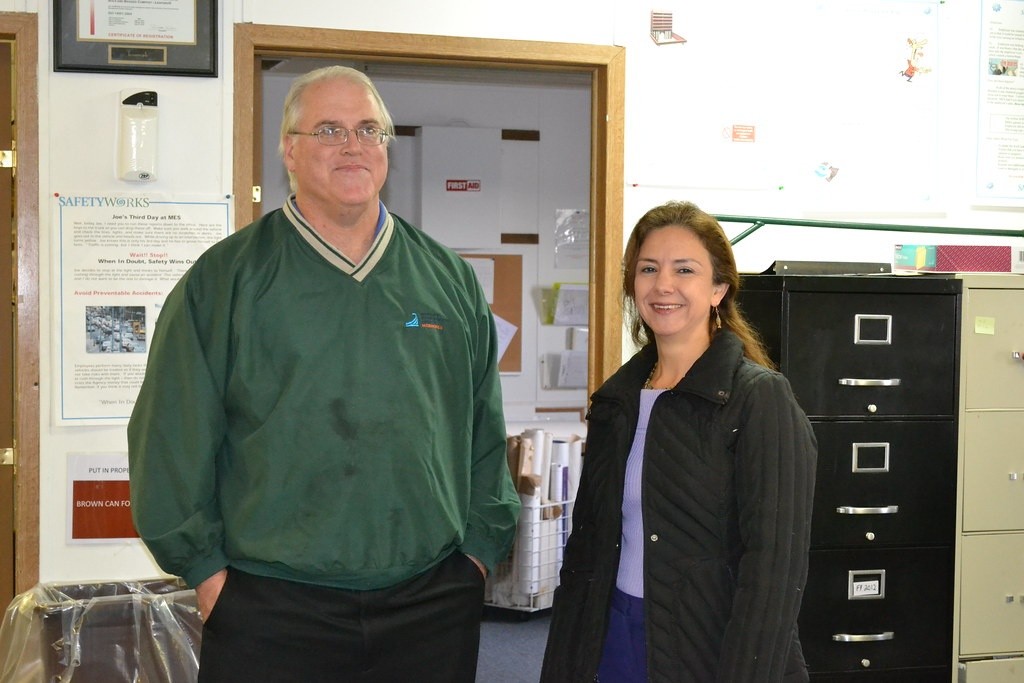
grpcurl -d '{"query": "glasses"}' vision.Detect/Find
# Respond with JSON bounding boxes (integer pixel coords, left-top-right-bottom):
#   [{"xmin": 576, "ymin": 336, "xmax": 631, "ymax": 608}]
[{"xmin": 289, "ymin": 124, "xmax": 392, "ymax": 145}]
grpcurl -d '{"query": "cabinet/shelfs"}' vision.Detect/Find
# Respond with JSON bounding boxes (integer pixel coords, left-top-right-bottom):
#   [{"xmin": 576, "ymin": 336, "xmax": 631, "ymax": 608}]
[
  {"xmin": 933, "ymin": 273, "xmax": 1024, "ymax": 683},
  {"xmin": 729, "ymin": 261, "xmax": 964, "ymax": 682}
]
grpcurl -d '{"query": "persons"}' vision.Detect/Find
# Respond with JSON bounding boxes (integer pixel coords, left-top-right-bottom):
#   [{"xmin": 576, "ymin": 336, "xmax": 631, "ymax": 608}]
[
  {"xmin": 537, "ymin": 198, "xmax": 816, "ymax": 682},
  {"xmin": 128, "ymin": 63, "xmax": 524, "ymax": 683}
]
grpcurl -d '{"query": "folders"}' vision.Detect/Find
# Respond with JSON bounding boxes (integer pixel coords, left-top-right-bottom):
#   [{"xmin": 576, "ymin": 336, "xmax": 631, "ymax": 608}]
[{"xmin": 757, "ymin": 260, "xmax": 891, "ymax": 275}]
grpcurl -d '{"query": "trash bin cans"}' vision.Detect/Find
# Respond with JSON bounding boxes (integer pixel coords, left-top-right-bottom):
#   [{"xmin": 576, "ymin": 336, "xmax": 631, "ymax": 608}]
[{"xmin": 32, "ymin": 574, "xmax": 204, "ymax": 682}]
